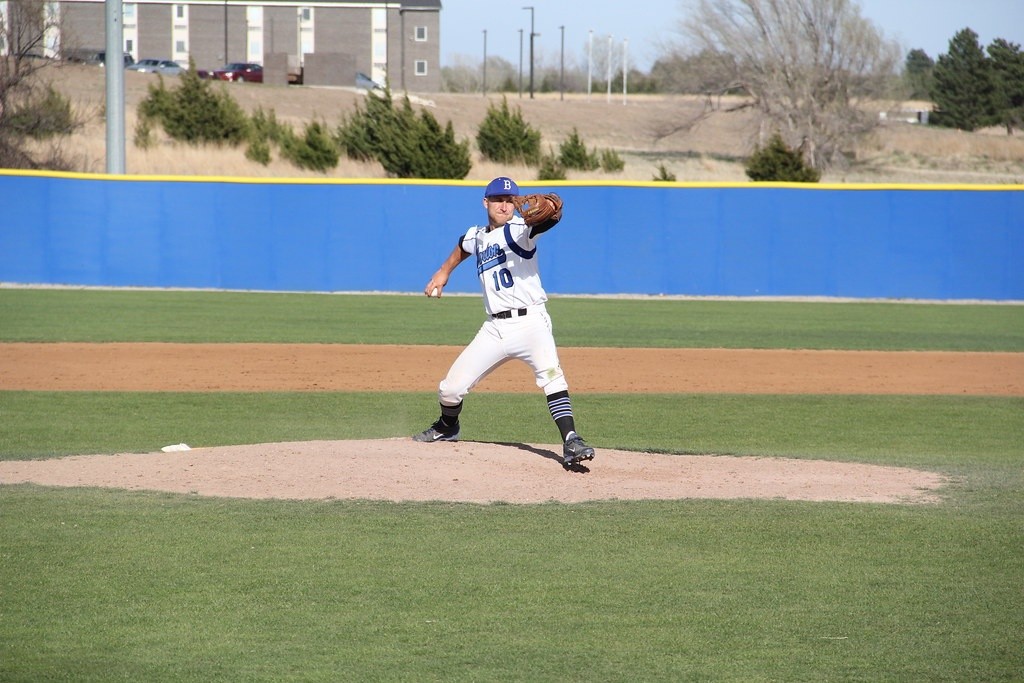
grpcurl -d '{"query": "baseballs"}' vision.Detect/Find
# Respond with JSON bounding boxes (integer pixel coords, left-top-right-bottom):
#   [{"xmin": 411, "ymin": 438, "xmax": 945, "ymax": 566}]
[{"xmin": 431, "ymin": 288, "xmax": 438, "ymax": 297}]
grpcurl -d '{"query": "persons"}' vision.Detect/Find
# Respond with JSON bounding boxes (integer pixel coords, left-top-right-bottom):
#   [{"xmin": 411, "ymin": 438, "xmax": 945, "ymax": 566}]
[{"xmin": 413, "ymin": 177, "xmax": 595, "ymax": 462}]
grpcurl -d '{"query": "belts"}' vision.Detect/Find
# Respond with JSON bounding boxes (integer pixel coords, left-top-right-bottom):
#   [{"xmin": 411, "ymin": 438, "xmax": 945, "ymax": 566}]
[{"xmin": 491, "ymin": 308, "xmax": 527, "ymax": 318}]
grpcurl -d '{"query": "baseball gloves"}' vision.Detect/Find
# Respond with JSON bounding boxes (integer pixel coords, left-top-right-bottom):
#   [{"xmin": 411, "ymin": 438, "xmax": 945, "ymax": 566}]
[{"xmin": 509, "ymin": 192, "xmax": 563, "ymax": 228}]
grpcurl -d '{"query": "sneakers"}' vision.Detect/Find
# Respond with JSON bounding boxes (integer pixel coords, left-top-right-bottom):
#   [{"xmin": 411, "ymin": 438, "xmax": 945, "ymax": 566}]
[
  {"xmin": 411, "ymin": 415, "xmax": 460, "ymax": 443},
  {"xmin": 563, "ymin": 433, "xmax": 596, "ymax": 466}
]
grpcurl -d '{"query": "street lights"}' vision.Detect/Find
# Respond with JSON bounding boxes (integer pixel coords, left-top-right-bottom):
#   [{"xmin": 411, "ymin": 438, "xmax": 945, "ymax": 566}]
[
  {"xmin": 558, "ymin": 25, "xmax": 564, "ymax": 100},
  {"xmin": 623, "ymin": 37, "xmax": 628, "ymax": 95},
  {"xmin": 607, "ymin": 35, "xmax": 613, "ymax": 94},
  {"xmin": 523, "ymin": 6, "xmax": 535, "ymax": 99},
  {"xmin": 482, "ymin": 29, "xmax": 487, "ymax": 96},
  {"xmin": 587, "ymin": 29, "xmax": 594, "ymax": 94},
  {"xmin": 518, "ymin": 29, "xmax": 524, "ymax": 97}
]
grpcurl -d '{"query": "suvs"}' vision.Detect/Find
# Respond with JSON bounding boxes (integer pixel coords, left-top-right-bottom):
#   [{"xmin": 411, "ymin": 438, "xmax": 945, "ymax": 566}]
[{"xmin": 85, "ymin": 49, "xmax": 134, "ymax": 68}]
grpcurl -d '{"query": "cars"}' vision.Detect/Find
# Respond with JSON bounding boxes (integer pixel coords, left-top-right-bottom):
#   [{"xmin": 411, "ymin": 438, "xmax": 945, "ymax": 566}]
[
  {"xmin": 354, "ymin": 70, "xmax": 384, "ymax": 92},
  {"xmin": 131, "ymin": 58, "xmax": 187, "ymax": 77},
  {"xmin": 198, "ymin": 62, "xmax": 262, "ymax": 83},
  {"xmin": 14, "ymin": 45, "xmax": 63, "ymax": 63}
]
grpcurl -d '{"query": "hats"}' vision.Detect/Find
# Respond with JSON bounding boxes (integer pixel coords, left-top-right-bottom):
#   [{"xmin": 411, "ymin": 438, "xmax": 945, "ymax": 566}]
[{"xmin": 485, "ymin": 176, "xmax": 519, "ymax": 198}]
